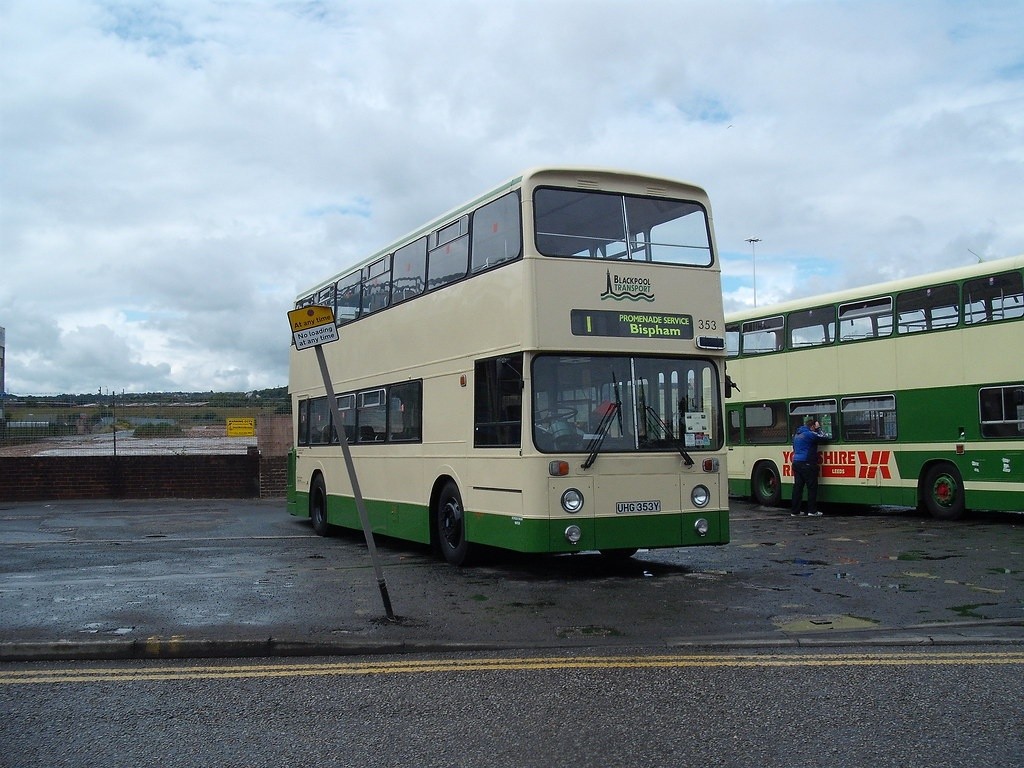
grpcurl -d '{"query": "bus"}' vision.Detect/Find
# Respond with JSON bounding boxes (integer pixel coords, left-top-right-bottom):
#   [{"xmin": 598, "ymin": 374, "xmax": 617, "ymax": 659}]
[
  {"xmin": 696, "ymin": 253, "xmax": 1024, "ymax": 527},
  {"xmin": 286, "ymin": 166, "xmax": 741, "ymax": 565}
]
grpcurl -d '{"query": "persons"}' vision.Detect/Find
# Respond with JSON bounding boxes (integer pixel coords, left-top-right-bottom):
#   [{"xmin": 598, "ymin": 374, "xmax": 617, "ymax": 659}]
[{"xmin": 790, "ymin": 416, "xmax": 828, "ymax": 518}]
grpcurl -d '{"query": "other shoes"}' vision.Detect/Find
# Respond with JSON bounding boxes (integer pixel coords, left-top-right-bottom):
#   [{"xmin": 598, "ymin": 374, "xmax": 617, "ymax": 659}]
[
  {"xmin": 808, "ymin": 511, "xmax": 823, "ymax": 516},
  {"xmin": 791, "ymin": 512, "xmax": 804, "ymax": 516}
]
